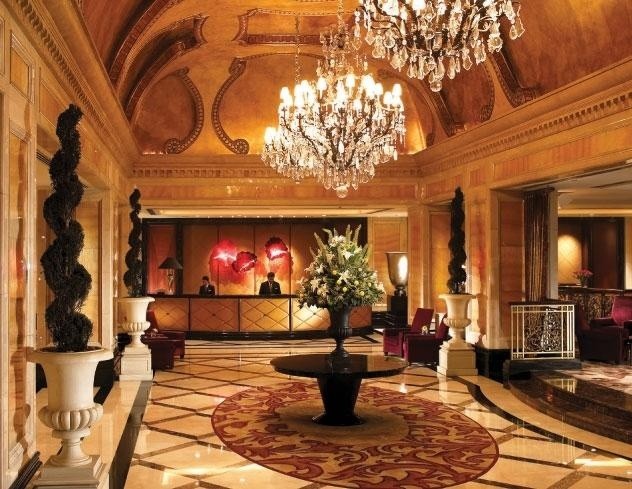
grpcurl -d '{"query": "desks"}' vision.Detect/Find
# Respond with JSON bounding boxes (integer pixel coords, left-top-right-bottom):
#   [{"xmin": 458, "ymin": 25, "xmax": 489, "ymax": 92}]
[
  {"xmin": 148, "ymin": 295, "xmax": 372, "ymax": 338},
  {"xmin": 271, "ymin": 354, "xmax": 406, "ymax": 425}
]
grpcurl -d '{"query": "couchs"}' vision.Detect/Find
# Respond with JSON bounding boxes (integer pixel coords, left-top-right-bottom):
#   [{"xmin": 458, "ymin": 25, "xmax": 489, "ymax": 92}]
[
  {"xmin": 577, "ymin": 319, "xmax": 629, "ymax": 364},
  {"xmin": 403, "ymin": 314, "xmax": 449, "ymax": 364},
  {"xmin": 138, "ymin": 339, "xmax": 174, "ymax": 369},
  {"xmin": 147, "ymin": 310, "xmax": 184, "ymax": 358},
  {"xmin": 383, "ymin": 309, "xmax": 434, "ymax": 359}
]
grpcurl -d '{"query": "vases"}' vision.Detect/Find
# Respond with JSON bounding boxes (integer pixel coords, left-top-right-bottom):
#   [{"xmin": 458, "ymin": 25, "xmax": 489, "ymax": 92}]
[
  {"xmin": 573, "ymin": 277, "xmax": 592, "ymax": 289},
  {"xmin": 298, "ymin": 304, "xmax": 385, "ymax": 358}
]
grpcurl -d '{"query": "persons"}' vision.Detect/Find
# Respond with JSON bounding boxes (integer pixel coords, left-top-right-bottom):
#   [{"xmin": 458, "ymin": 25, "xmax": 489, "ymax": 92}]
[
  {"xmin": 199, "ymin": 276, "xmax": 216, "ymax": 294},
  {"xmin": 260, "ymin": 272, "xmax": 281, "ymax": 295}
]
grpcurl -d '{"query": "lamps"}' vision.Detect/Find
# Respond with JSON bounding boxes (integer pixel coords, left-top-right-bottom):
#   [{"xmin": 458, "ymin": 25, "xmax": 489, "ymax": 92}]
[
  {"xmin": 158, "ymin": 257, "xmax": 183, "ymax": 296},
  {"xmin": 386, "ymin": 252, "xmax": 408, "ymax": 297},
  {"xmin": 356, "ymin": 1, "xmax": 524, "ymax": 92},
  {"xmin": 261, "ymin": 0, "xmax": 405, "ymax": 198}
]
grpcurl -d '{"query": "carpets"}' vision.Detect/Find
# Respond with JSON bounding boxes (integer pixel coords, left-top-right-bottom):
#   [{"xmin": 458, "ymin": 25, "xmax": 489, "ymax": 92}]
[{"xmin": 212, "ymin": 380, "xmax": 498, "ymax": 489}]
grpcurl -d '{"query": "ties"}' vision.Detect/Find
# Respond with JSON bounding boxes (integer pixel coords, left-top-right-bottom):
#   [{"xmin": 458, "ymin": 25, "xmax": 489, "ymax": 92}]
[{"xmin": 205, "ymin": 287, "xmax": 207, "ymax": 295}]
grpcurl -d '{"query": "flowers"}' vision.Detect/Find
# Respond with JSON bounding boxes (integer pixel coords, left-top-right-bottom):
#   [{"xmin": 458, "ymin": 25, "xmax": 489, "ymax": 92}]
[
  {"xmin": 301, "ymin": 222, "xmax": 386, "ymax": 304},
  {"xmin": 572, "ymin": 269, "xmax": 591, "ymax": 276}
]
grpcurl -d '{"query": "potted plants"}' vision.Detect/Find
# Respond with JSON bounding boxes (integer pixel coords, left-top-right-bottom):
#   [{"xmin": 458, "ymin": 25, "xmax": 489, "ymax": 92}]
[
  {"xmin": 439, "ymin": 187, "xmax": 478, "ymax": 376},
  {"xmin": 118, "ymin": 189, "xmax": 155, "ymax": 381},
  {"xmin": 25, "ymin": 104, "xmax": 114, "ymax": 489}
]
